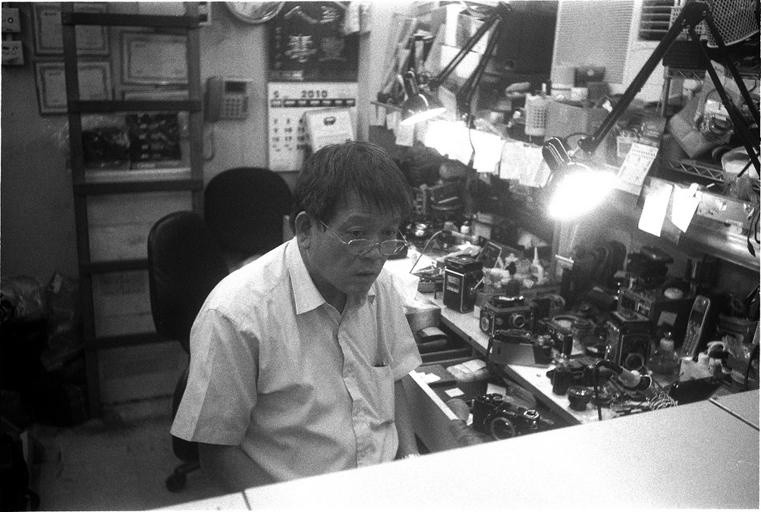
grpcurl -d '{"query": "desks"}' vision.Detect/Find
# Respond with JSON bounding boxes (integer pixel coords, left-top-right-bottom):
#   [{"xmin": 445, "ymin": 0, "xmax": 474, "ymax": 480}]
[{"xmin": 161, "ymin": 236, "xmax": 760, "ymax": 509}]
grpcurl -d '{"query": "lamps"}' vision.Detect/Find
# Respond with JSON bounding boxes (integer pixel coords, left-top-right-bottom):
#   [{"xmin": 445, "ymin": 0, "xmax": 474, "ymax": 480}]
[
  {"xmin": 538, "ymin": 0, "xmax": 759, "ymax": 228},
  {"xmin": 397, "ymin": 1, "xmax": 512, "ymax": 131}
]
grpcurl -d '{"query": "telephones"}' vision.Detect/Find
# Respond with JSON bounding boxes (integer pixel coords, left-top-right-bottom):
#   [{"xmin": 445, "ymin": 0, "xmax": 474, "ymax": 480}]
[
  {"xmin": 204, "ymin": 76, "xmax": 250, "ymax": 123},
  {"xmin": 678, "ymin": 294, "xmax": 714, "ymax": 363}
]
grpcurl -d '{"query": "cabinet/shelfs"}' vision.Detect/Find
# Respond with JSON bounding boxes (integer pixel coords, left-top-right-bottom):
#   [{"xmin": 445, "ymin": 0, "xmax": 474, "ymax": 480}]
[{"xmin": 56, "ymin": 4, "xmax": 208, "ymax": 419}]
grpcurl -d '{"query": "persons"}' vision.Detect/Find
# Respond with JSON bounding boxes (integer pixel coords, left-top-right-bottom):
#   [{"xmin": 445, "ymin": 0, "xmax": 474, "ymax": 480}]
[{"xmin": 172, "ymin": 140, "xmax": 423, "ymax": 490}]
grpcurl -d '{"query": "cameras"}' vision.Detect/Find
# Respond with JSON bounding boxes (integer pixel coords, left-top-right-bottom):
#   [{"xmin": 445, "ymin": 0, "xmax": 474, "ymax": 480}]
[{"xmin": 472, "ymin": 297, "xmax": 650, "ymax": 440}]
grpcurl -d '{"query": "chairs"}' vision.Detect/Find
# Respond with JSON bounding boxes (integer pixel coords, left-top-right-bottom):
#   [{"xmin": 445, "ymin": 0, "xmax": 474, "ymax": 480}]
[{"xmin": 146, "ymin": 163, "xmax": 295, "ymax": 495}]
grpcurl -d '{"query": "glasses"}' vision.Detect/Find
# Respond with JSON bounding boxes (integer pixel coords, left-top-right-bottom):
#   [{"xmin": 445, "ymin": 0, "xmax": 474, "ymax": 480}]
[{"xmin": 320, "ymin": 220, "xmax": 407, "ymax": 256}]
